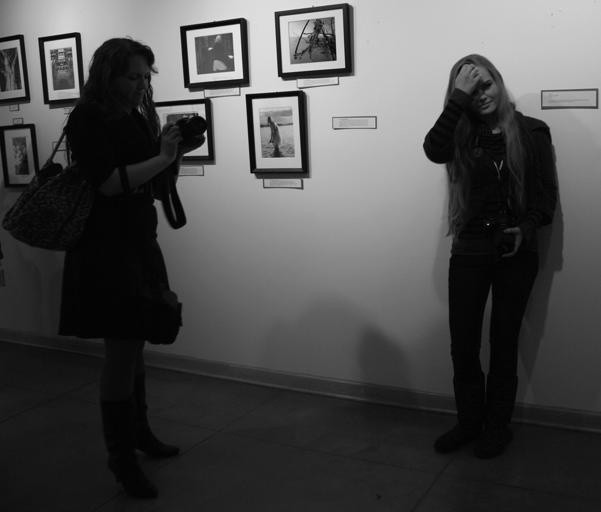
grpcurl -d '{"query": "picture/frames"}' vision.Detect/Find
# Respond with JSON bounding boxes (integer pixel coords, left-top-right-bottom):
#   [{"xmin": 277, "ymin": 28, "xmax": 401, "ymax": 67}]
[
  {"xmin": 274, "ymin": 5, "xmax": 352, "ymax": 78},
  {"xmin": 38, "ymin": 31, "xmax": 85, "ymax": 104},
  {"xmin": 3, "ymin": 124, "xmax": 40, "ymax": 189},
  {"xmin": 178, "ymin": 18, "xmax": 247, "ymax": 88},
  {"xmin": 245, "ymin": 90, "xmax": 310, "ymax": 174},
  {"xmin": 154, "ymin": 99, "xmax": 214, "ymax": 161},
  {"xmin": 1, "ymin": 34, "xmax": 31, "ymax": 104}
]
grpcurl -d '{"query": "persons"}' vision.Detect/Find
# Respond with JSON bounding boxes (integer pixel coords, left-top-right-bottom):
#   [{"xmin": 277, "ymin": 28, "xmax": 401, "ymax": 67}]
[
  {"xmin": 420, "ymin": 51, "xmax": 558, "ymax": 459},
  {"xmin": 57, "ymin": 36, "xmax": 193, "ymax": 501},
  {"xmin": 266, "ymin": 116, "xmax": 283, "ymax": 156}
]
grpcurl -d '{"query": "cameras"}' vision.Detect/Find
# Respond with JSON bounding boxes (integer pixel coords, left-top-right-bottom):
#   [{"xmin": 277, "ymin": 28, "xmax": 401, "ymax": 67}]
[{"xmin": 163, "ymin": 111, "xmax": 208, "ymax": 139}]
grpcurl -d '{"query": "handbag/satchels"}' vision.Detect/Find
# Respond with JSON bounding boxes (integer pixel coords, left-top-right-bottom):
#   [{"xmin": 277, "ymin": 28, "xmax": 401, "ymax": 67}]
[{"xmin": 1, "ymin": 131, "xmax": 99, "ymax": 252}]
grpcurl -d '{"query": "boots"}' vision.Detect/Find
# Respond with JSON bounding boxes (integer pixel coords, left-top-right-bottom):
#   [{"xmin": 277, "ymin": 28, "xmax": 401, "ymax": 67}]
[
  {"xmin": 432, "ymin": 371, "xmax": 486, "ymax": 453},
  {"xmin": 470, "ymin": 372, "xmax": 518, "ymax": 458},
  {"xmin": 137, "ymin": 374, "xmax": 180, "ymax": 459},
  {"xmin": 100, "ymin": 399, "xmax": 159, "ymax": 499}
]
